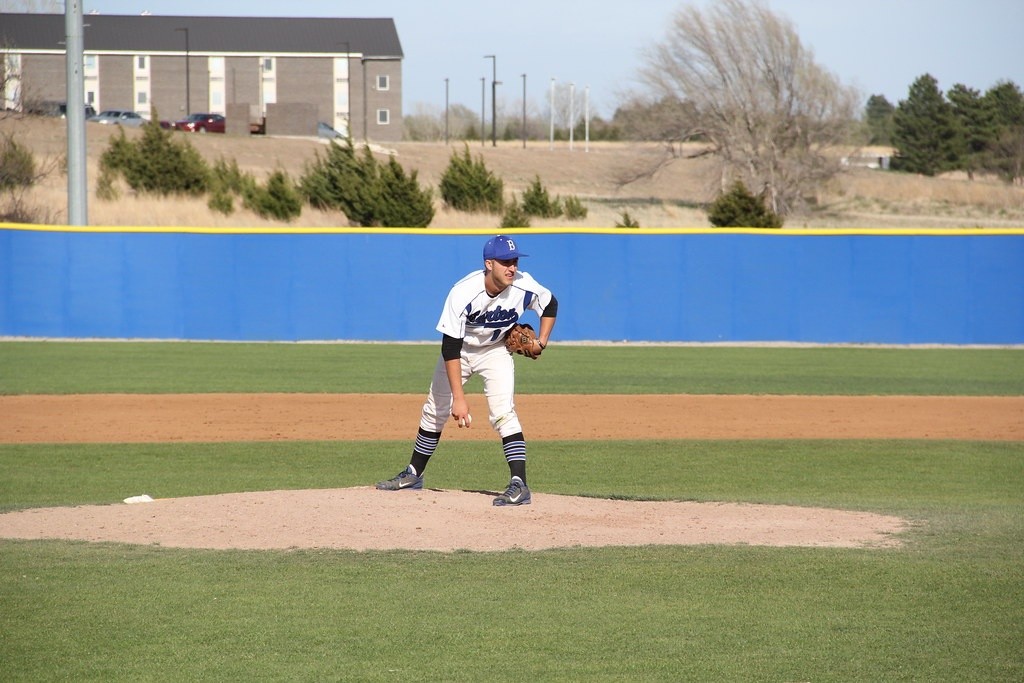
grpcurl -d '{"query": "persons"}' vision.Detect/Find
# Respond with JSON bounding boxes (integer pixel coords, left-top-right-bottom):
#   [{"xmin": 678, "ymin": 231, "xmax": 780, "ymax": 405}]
[{"xmin": 375, "ymin": 235, "xmax": 558, "ymax": 509}]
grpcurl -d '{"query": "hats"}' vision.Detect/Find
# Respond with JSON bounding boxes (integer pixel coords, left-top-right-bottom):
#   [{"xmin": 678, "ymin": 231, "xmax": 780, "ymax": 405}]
[{"xmin": 483, "ymin": 234, "xmax": 530, "ymax": 261}]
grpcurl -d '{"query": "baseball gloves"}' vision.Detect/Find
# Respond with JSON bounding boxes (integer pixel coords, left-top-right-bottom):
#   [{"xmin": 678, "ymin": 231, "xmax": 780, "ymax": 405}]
[{"xmin": 503, "ymin": 322, "xmax": 544, "ymax": 360}]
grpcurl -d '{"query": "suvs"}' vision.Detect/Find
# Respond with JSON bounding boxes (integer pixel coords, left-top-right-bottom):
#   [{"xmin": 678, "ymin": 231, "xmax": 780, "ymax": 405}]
[{"xmin": 27, "ymin": 100, "xmax": 96, "ymax": 122}]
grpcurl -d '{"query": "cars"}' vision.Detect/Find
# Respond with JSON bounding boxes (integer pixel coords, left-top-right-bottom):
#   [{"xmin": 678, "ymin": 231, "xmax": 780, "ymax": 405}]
[
  {"xmin": 318, "ymin": 121, "xmax": 348, "ymax": 136},
  {"xmin": 88, "ymin": 110, "xmax": 146, "ymax": 127},
  {"xmin": 173, "ymin": 113, "xmax": 224, "ymax": 134},
  {"xmin": 147, "ymin": 120, "xmax": 170, "ymax": 131}
]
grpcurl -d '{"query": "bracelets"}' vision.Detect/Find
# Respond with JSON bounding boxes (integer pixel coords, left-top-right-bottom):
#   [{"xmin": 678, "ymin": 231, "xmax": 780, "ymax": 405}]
[{"xmin": 536, "ymin": 337, "xmax": 545, "ymax": 350}]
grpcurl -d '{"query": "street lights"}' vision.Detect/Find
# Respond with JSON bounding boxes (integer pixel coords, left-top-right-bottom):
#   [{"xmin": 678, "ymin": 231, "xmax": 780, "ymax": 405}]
[
  {"xmin": 480, "ymin": 78, "xmax": 485, "ymax": 145},
  {"xmin": 492, "ymin": 81, "xmax": 503, "ymax": 141},
  {"xmin": 569, "ymin": 82, "xmax": 576, "ymax": 150},
  {"xmin": 444, "ymin": 77, "xmax": 450, "ymax": 144},
  {"xmin": 483, "ymin": 55, "xmax": 496, "ymax": 147},
  {"xmin": 174, "ymin": 26, "xmax": 190, "ymax": 119},
  {"xmin": 549, "ymin": 77, "xmax": 556, "ymax": 151},
  {"xmin": 520, "ymin": 74, "xmax": 527, "ymax": 148},
  {"xmin": 584, "ymin": 84, "xmax": 590, "ymax": 154}
]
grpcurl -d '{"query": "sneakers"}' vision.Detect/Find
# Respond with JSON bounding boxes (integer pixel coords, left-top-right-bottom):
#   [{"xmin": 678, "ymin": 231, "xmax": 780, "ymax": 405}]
[
  {"xmin": 376, "ymin": 463, "xmax": 424, "ymax": 491},
  {"xmin": 493, "ymin": 475, "xmax": 531, "ymax": 506}
]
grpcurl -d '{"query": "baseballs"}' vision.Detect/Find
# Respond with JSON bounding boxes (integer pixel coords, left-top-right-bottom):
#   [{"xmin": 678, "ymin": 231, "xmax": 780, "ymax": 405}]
[{"xmin": 459, "ymin": 414, "xmax": 473, "ymax": 427}]
